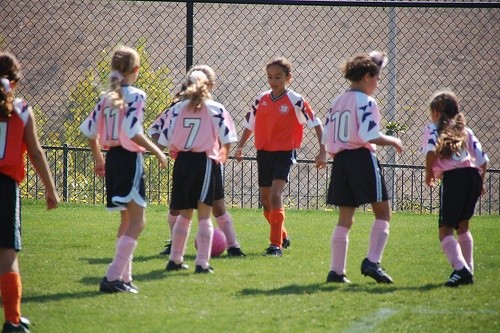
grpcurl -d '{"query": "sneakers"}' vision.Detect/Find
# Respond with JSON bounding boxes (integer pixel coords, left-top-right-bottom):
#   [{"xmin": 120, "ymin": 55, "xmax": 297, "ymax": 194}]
[
  {"xmin": 164, "ymin": 260, "xmax": 190, "ymax": 272},
  {"xmin": 326, "ymin": 271, "xmax": 351, "ymax": 284},
  {"xmin": 100, "ymin": 278, "xmax": 140, "ymax": 295},
  {"xmin": 361, "ymin": 258, "xmax": 394, "ymax": 284},
  {"xmin": 194, "ymin": 265, "xmax": 215, "ymax": 274},
  {"xmin": 159, "ymin": 242, "xmax": 172, "ymax": 255},
  {"xmin": 445, "ymin": 266, "xmax": 474, "ymax": 288},
  {"xmin": 262, "ymin": 245, "xmax": 282, "ymax": 257},
  {"xmin": 3, "ymin": 318, "xmax": 31, "ymax": 333},
  {"xmin": 227, "ymin": 246, "xmax": 246, "ymax": 258},
  {"xmin": 283, "ymin": 237, "xmax": 291, "ymax": 249}
]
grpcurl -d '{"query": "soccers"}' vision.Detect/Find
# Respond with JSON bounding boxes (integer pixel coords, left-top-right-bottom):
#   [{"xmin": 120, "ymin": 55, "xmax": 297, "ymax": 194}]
[{"xmin": 195, "ymin": 229, "xmax": 227, "ymax": 259}]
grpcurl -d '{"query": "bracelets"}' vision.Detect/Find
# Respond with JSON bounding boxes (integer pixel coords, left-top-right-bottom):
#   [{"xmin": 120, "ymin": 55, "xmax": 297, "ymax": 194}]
[{"xmin": 235, "ymin": 148, "xmax": 243, "ymax": 151}]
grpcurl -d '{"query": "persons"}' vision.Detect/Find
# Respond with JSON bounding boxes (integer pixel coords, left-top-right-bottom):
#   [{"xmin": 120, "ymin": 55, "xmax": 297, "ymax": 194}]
[
  {"xmin": 0, "ymin": 51, "xmax": 62, "ymax": 333},
  {"xmin": 233, "ymin": 58, "xmax": 329, "ymax": 256},
  {"xmin": 322, "ymin": 54, "xmax": 404, "ymax": 284},
  {"xmin": 80, "ymin": 46, "xmax": 167, "ymax": 295},
  {"xmin": 150, "ymin": 65, "xmax": 246, "ymax": 273},
  {"xmin": 424, "ymin": 91, "xmax": 488, "ymax": 288}
]
